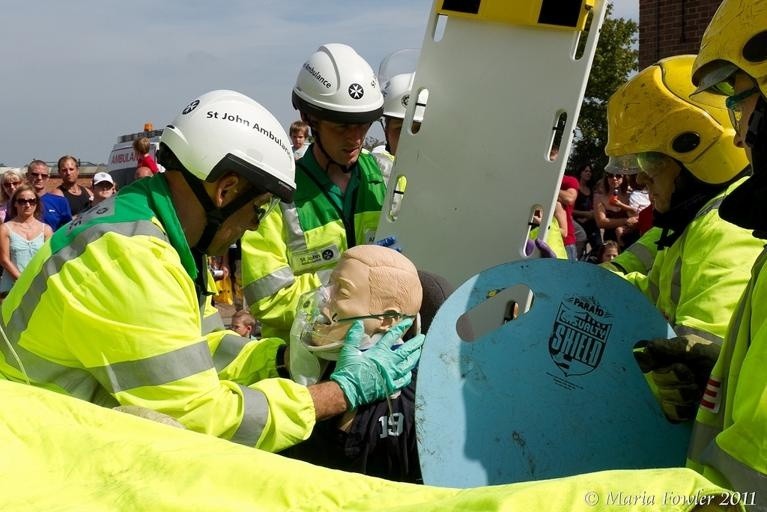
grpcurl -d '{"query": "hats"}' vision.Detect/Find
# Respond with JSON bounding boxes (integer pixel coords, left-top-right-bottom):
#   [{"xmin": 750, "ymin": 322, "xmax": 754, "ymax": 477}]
[{"xmin": 93, "ymin": 172, "xmax": 113, "ymax": 185}]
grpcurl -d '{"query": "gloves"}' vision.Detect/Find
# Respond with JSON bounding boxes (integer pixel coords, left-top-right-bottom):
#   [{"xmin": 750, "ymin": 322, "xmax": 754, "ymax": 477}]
[{"xmin": 633, "ymin": 334, "xmax": 720, "ymax": 424}]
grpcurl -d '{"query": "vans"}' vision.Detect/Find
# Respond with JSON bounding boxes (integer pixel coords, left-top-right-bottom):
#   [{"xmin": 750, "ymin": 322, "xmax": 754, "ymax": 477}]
[{"xmin": 106, "ymin": 125, "xmax": 163, "ymax": 190}]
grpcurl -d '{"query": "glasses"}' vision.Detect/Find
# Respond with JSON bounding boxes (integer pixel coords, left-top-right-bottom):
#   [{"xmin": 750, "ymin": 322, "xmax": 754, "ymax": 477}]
[
  {"xmin": 725, "ymin": 86, "xmax": 758, "ymax": 135},
  {"xmin": 31, "ymin": 173, "xmax": 48, "ymax": 178},
  {"xmin": 16, "ymin": 199, "xmax": 36, "ymax": 205},
  {"xmin": 608, "ymin": 174, "xmax": 623, "ymax": 178},
  {"xmin": 247, "ymin": 198, "xmax": 268, "ymax": 225},
  {"xmin": 3, "ymin": 182, "xmax": 20, "ymax": 186}
]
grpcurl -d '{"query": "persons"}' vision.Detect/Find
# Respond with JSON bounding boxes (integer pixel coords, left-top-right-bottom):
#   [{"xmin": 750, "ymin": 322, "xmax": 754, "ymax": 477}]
[
  {"xmin": 241, "ymin": 41, "xmax": 386, "ymax": 347},
  {"xmin": 273, "ymin": 241, "xmax": 424, "ymax": 488},
  {"xmin": 0, "ymin": 87, "xmax": 425, "ymax": 453},
  {"xmin": 0, "ymin": 72, "xmax": 429, "ymax": 344},
  {"xmin": 594, "ymin": 54, "xmax": 750, "ymax": 347},
  {"xmin": 631, "ymin": 1, "xmax": 766, "ymax": 512},
  {"xmin": 501, "ymin": 121, "xmax": 660, "ymax": 324}
]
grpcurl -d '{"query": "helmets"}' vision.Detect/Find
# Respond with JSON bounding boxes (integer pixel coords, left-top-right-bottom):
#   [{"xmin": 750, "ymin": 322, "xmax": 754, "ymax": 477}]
[
  {"xmin": 292, "ymin": 44, "xmax": 384, "ymax": 123},
  {"xmin": 604, "ymin": 54, "xmax": 750, "ymax": 183},
  {"xmin": 689, "ymin": 1, "xmax": 766, "ymax": 101},
  {"xmin": 382, "ymin": 71, "xmax": 429, "ymax": 124},
  {"xmin": 157, "ymin": 90, "xmax": 296, "ymax": 203}
]
